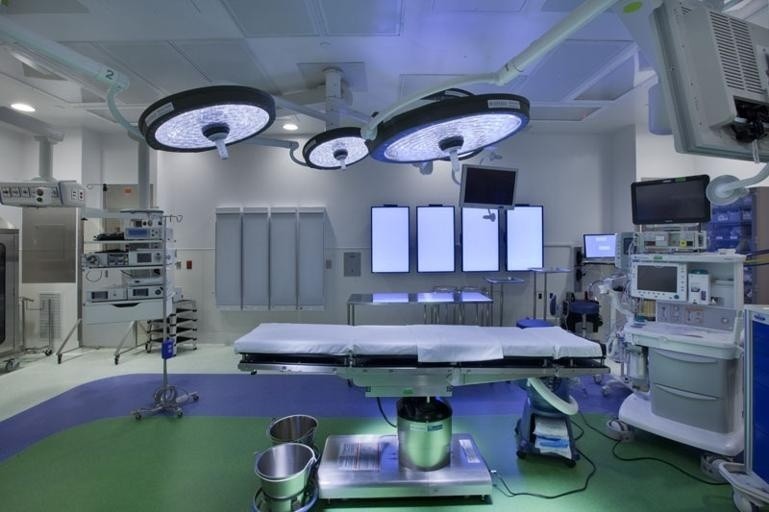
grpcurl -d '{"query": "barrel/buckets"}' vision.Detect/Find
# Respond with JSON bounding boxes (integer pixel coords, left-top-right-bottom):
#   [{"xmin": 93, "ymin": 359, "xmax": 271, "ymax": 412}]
[
  {"xmin": 266, "ymin": 413, "xmax": 319, "ymax": 449},
  {"xmin": 253, "ymin": 442, "xmax": 318, "ymax": 512}
]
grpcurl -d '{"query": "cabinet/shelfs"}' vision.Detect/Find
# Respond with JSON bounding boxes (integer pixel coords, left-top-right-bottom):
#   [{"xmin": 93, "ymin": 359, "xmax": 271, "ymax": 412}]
[{"xmin": 716, "ymin": 304, "xmax": 769, "ymax": 512}]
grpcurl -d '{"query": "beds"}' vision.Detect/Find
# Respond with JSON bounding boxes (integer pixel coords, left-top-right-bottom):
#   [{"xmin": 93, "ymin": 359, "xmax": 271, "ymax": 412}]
[
  {"xmin": 345, "ymin": 290, "xmax": 494, "ymax": 326},
  {"xmin": 233, "ymin": 322, "xmax": 610, "ymax": 505}
]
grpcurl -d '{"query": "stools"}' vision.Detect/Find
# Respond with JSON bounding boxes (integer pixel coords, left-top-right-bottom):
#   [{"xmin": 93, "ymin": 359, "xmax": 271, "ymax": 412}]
[
  {"xmin": 569, "ymin": 301, "xmax": 606, "ymax": 369},
  {"xmin": 516, "ymin": 318, "xmax": 560, "ymax": 393}
]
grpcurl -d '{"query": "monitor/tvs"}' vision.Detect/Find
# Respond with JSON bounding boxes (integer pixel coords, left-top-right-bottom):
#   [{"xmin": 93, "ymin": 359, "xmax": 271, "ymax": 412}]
[
  {"xmin": 649, "ymin": 1, "xmax": 769, "ymax": 162},
  {"xmin": 584, "ymin": 232, "xmax": 614, "ymax": 259},
  {"xmin": 458, "ymin": 162, "xmax": 517, "ymax": 211},
  {"xmin": 630, "ymin": 174, "xmax": 710, "ymax": 225}
]
